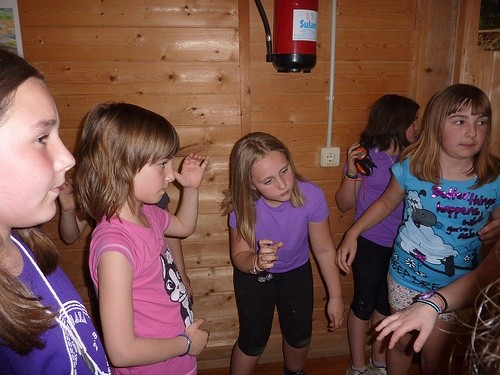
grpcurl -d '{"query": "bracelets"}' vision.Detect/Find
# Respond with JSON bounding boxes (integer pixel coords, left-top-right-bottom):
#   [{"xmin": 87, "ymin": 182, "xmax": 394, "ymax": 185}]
[
  {"xmin": 342, "ymin": 171, "xmax": 366, "ymax": 183},
  {"xmin": 250, "ymin": 253, "xmax": 273, "ymax": 283},
  {"xmin": 177, "ymin": 334, "xmax": 191, "ymax": 357}
]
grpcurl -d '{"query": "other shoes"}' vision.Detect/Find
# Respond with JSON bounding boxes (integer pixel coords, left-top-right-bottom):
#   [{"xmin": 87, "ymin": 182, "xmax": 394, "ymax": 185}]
[
  {"xmin": 346, "ymin": 360, "xmax": 369, "ymax": 375},
  {"xmin": 367, "ymin": 360, "xmax": 387, "ymax": 375}
]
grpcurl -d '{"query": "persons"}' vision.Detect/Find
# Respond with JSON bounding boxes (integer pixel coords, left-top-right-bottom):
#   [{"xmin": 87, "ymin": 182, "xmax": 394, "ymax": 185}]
[
  {"xmin": 335, "ymin": 95, "xmax": 421, "ymax": 375},
  {"xmin": 0, "ymin": 46, "xmax": 112, "ymax": 375},
  {"xmin": 375, "ymin": 234, "xmax": 500, "ymax": 353},
  {"xmin": 219, "ymin": 131, "xmax": 345, "ymax": 375},
  {"xmin": 333, "ymin": 84, "xmax": 500, "ymax": 375},
  {"xmin": 73, "ymin": 102, "xmax": 211, "ymax": 375},
  {"xmin": 58, "ymin": 173, "xmax": 193, "ymax": 304}
]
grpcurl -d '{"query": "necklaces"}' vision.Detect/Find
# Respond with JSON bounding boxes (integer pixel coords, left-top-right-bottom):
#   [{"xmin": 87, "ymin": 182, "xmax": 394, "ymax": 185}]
[{"xmin": 384, "ymin": 151, "xmax": 395, "ymax": 165}]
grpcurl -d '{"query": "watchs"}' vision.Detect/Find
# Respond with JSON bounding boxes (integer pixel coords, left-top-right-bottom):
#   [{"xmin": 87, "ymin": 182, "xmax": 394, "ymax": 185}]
[{"xmin": 416, "ymin": 291, "xmax": 448, "ymax": 314}]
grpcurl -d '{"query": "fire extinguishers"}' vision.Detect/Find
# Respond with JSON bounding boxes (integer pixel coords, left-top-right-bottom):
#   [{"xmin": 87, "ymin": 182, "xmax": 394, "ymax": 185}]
[{"xmin": 254, "ymin": 0, "xmax": 318, "ymax": 72}]
